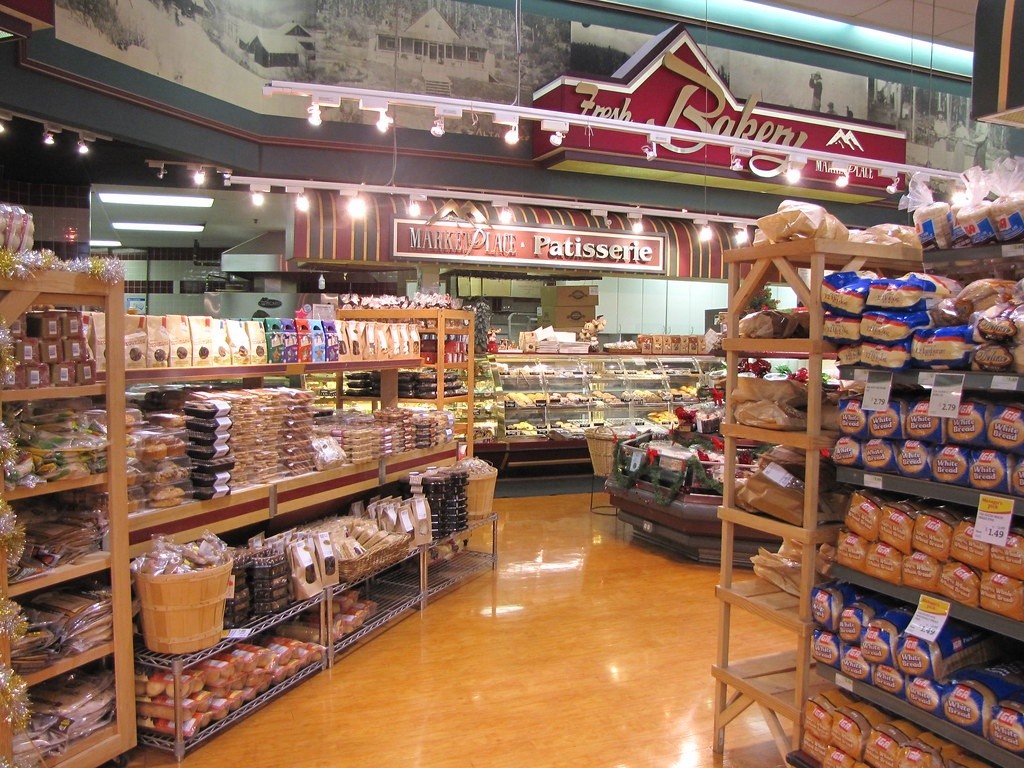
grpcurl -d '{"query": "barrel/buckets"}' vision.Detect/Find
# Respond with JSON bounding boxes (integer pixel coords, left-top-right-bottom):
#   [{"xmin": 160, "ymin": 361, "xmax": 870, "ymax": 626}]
[
  {"xmin": 132, "ymin": 554, "xmax": 234, "ymax": 654},
  {"xmin": 462, "ymin": 460, "xmax": 499, "ymax": 520}
]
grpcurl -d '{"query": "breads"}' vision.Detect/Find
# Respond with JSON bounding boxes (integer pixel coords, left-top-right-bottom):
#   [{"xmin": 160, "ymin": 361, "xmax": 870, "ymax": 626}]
[
  {"xmin": 800, "ymin": 192, "xmax": 1024, "ymax": 768},
  {"xmin": 87, "ymin": 388, "xmax": 376, "ymax": 739}
]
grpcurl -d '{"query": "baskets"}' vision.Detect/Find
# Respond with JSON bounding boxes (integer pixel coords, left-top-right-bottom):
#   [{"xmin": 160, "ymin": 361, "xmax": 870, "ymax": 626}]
[{"xmin": 587, "ymin": 428, "xmax": 638, "ymax": 477}]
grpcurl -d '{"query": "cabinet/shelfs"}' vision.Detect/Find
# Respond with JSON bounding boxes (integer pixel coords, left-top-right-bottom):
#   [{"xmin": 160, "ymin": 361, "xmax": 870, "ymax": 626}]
[
  {"xmin": 335, "ymin": 306, "xmax": 475, "ymax": 458},
  {"xmin": 98, "ymin": 512, "xmax": 499, "ymax": 760},
  {"xmin": 786, "ymin": 245, "xmax": 1024, "ymax": 768},
  {"xmin": 66, "ymin": 357, "xmax": 457, "ymax": 563},
  {"xmin": 0, "ymin": 265, "xmax": 141, "ymax": 768},
  {"xmin": 717, "ymin": 240, "xmax": 919, "ymax": 768}
]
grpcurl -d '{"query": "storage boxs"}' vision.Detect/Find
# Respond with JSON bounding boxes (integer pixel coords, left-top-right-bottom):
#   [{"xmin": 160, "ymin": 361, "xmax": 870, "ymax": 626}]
[
  {"xmin": 6, "ymin": 309, "xmax": 468, "ymax": 626},
  {"xmin": 537, "ymin": 306, "xmax": 596, "ymax": 327},
  {"xmin": 554, "ymin": 327, "xmax": 584, "ymax": 341},
  {"xmin": 536, "ymin": 325, "xmax": 554, "ymax": 340},
  {"xmin": 637, "ymin": 334, "xmax": 708, "ymax": 355},
  {"xmin": 540, "ymin": 285, "xmax": 599, "ymax": 306},
  {"xmin": 524, "ymin": 332, "xmax": 536, "ymax": 353},
  {"xmin": 547, "ymin": 332, "xmax": 576, "ymax": 341},
  {"xmin": 224, "ymin": 547, "xmax": 291, "ymax": 628},
  {"xmin": 559, "ymin": 342, "xmax": 591, "ymax": 354},
  {"xmin": 519, "ymin": 331, "xmax": 525, "ymax": 349},
  {"xmin": 536, "ymin": 340, "xmax": 559, "ymax": 354}
]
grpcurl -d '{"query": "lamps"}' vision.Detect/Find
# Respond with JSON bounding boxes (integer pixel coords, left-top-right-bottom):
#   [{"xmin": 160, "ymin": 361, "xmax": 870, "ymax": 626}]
[
  {"xmin": 626, "ymin": 213, "xmax": 643, "ymax": 232},
  {"xmin": 491, "ymin": 201, "xmax": 512, "ymax": 223},
  {"xmin": 42, "ymin": 123, "xmax": 62, "ymax": 145},
  {"xmin": 185, "ymin": 166, "xmax": 206, "ymax": 186},
  {"xmin": 540, "ymin": 120, "xmax": 569, "ymax": 146},
  {"xmin": 148, "ymin": 163, "xmax": 167, "ymax": 180},
  {"xmin": 359, "ymin": 99, "xmax": 394, "ymax": 134},
  {"xmin": 828, "ymin": 161, "xmax": 851, "ymax": 187},
  {"xmin": 307, "ymin": 96, "xmax": 342, "ymax": 126},
  {"xmin": 430, "ymin": 106, "xmax": 463, "ymax": 137},
  {"xmin": 783, "ymin": 154, "xmax": 808, "ymax": 183},
  {"xmin": 733, "ymin": 223, "xmax": 749, "ymax": 245},
  {"xmin": 409, "ymin": 195, "xmax": 427, "ymax": 215},
  {"xmin": 590, "ymin": 210, "xmax": 613, "ymax": 226},
  {"xmin": 285, "ymin": 186, "xmax": 309, "ymax": 212},
  {"xmin": 216, "ymin": 168, "xmax": 233, "ymax": 186},
  {"xmin": 77, "ymin": 132, "xmax": 96, "ymax": 154},
  {"xmin": 491, "ymin": 112, "xmax": 520, "ymax": 144},
  {"xmin": 878, "ymin": 169, "xmax": 900, "ymax": 194},
  {"xmin": 641, "ymin": 133, "xmax": 671, "ymax": 161},
  {"xmin": 340, "ymin": 190, "xmax": 365, "ymax": 217},
  {"xmin": 249, "ymin": 184, "xmax": 271, "ymax": 206},
  {"xmin": 729, "ymin": 146, "xmax": 753, "ymax": 171},
  {"xmin": 693, "ymin": 218, "xmax": 712, "ymax": 241},
  {"xmin": 0, "ymin": 110, "xmax": 13, "ymax": 132}
]
